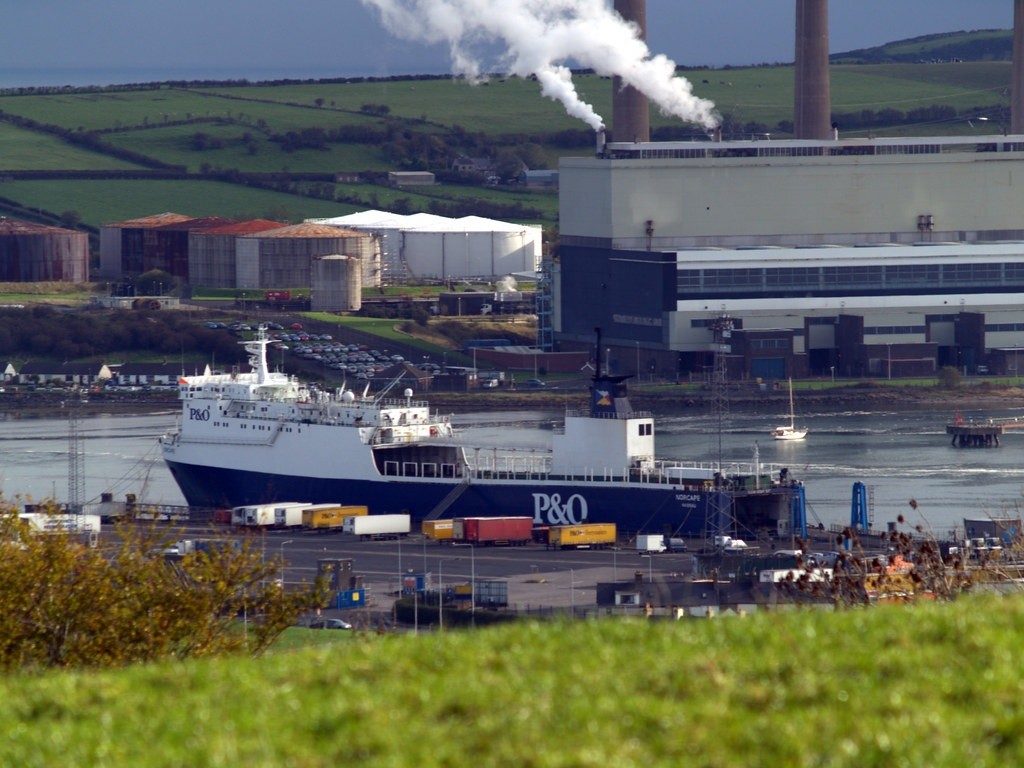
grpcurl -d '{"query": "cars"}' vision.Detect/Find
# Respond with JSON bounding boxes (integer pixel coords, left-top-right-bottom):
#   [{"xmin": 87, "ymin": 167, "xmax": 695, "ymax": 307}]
[{"xmin": 210, "ymin": 317, "xmax": 415, "ymax": 380}]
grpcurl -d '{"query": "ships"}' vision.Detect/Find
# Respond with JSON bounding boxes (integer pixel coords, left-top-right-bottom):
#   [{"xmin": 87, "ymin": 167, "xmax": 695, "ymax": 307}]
[{"xmin": 161, "ymin": 321, "xmax": 792, "ymax": 540}]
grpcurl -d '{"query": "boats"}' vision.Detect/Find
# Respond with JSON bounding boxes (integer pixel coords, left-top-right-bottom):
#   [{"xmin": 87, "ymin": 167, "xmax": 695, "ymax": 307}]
[{"xmin": 773, "ymin": 424, "xmax": 810, "ymax": 441}]
